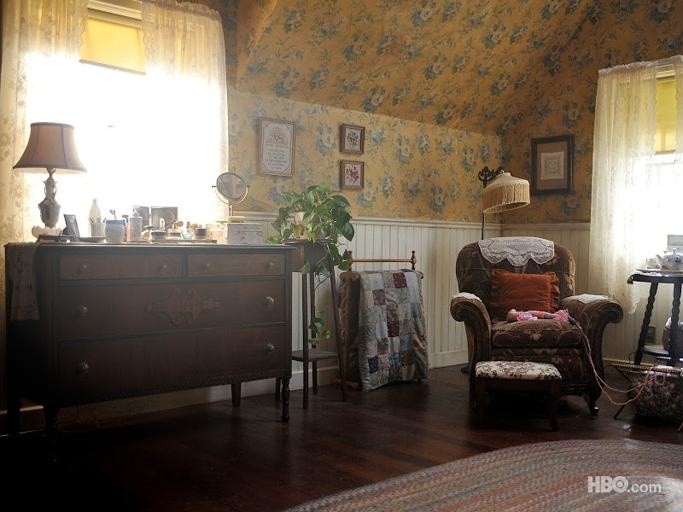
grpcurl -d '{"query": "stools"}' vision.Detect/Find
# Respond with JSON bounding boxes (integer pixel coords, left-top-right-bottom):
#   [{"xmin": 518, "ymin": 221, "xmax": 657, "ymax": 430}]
[{"xmin": 475, "ymin": 361, "xmax": 562, "ymax": 432}]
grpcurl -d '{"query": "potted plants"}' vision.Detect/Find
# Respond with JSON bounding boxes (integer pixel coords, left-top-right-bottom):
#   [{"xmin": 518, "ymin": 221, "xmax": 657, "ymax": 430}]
[{"xmin": 267, "ymin": 183, "xmax": 354, "ymax": 340}]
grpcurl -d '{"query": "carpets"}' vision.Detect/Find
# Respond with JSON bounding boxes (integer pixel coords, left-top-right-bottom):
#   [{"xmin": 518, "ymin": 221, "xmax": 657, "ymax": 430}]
[{"xmin": 286, "ymin": 439, "xmax": 683, "ymax": 512}]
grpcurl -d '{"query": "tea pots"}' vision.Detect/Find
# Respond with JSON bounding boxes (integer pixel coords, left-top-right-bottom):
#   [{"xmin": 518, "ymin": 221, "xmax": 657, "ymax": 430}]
[
  {"xmin": 655, "ymin": 247, "xmax": 682, "ymax": 270},
  {"xmin": 643, "ymin": 257, "xmax": 660, "ymax": 270}
]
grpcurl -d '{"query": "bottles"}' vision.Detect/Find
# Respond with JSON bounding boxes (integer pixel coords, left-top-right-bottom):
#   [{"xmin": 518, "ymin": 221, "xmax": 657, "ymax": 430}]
[{"xmin": 87, "ymin": 199, "xmax": 101, "ymax": 237}]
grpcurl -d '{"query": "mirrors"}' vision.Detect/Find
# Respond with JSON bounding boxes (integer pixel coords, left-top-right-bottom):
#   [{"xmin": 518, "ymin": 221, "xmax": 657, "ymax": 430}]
[{"xmin": 211, "ymin": 172, "xmax": 249, "ymax": 223}]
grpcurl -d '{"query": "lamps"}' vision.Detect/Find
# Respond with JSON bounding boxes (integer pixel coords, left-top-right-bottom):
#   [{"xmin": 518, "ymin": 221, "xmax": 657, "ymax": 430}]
[
  {"xmin": 478, "ymin": 166, "xmax": 530, "ymax": 240},
  {"xmin": 12, "ymin": 122, "xmax": 86, "ymax": 238}
]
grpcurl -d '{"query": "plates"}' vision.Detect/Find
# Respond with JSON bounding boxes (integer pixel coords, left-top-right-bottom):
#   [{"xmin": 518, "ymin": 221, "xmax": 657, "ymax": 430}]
[
  {"xmin": 636, "ymin": 267, "xmax": 662, "ymax": 273},
  {"xmin": 654, "ymin": 267, "xmax": 681, "ymax": 277}
]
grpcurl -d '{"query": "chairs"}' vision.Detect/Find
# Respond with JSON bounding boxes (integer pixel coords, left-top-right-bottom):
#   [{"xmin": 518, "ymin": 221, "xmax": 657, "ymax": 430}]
[{"xmin": 450, "ymin": 236, "xmax": 623, "ymax": 414}]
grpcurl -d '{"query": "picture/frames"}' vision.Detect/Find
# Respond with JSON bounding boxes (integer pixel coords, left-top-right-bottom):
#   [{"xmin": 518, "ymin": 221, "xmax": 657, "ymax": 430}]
[
  {"xmin": 340, "ymin": 160, "xmax": 364, "ymax": 190},
  {"xmin": 257, "ymin": 117, "xmax": 296, "ymax": 178},
  {"xmin": 339, "ymin": 123, "xmax": 365, "ymax": 155},
  {"xmin": 530, "ymin": 134, "xmax": 575, "ymax": 196}
]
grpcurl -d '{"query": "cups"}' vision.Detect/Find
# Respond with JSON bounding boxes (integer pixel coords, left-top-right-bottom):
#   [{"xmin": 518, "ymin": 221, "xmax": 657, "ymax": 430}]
[
  {"xmin": 146, "ymin": 223, "xmax": 209, "ymax": 242},
  {"xmin": 125, "ymin": 217, "xmax": 144, "ymax": 244},
  {"xmin": 103, "ymin": 220, "xmax": 126, "ymax": 244}
]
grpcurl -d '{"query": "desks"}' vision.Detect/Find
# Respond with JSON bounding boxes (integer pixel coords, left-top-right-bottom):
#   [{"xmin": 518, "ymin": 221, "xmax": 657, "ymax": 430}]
[
  {"xmin": 626, "ymin": 272, "xmax": 683, "ymax": 372},
  {"xmin": 4, "ymin": 242, "xmax": 297, "ymax": 462}
]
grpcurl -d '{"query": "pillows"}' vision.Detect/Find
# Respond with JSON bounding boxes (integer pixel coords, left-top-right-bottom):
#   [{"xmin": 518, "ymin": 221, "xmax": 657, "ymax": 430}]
[{"xmin": 490, "ymin": 269, "xmax": 560, "ymax": 321}]
